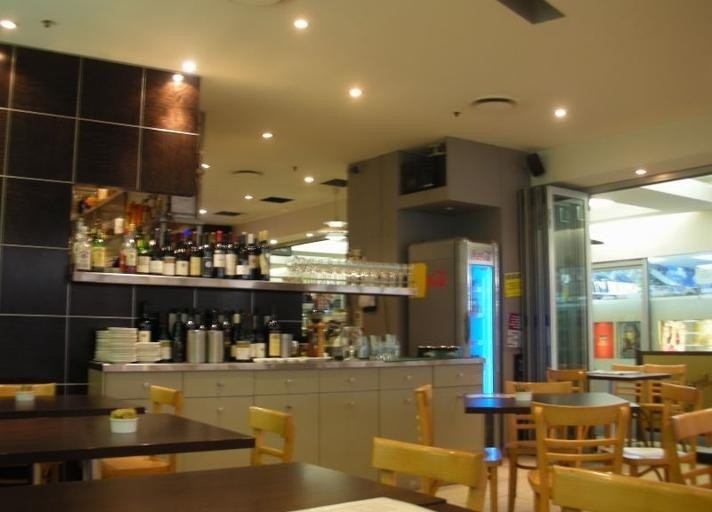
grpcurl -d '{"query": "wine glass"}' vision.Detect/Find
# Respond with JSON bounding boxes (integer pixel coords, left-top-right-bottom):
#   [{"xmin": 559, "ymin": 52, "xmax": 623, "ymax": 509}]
[{"xmin": 285, "ymin": 255, "xmax": 415, "ymax": 288}]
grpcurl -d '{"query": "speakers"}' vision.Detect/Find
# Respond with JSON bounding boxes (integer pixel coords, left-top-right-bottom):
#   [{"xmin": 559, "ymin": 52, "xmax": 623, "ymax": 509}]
[{"xmin": 527, "ymin": 153, "xmax": 544, "ymax": 176}]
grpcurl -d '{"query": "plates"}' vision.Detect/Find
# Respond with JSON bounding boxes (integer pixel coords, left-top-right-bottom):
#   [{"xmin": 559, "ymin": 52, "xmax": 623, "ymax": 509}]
[{"xmin": 92, "ymin": 326, "xmax": 171, "ymax": 364}]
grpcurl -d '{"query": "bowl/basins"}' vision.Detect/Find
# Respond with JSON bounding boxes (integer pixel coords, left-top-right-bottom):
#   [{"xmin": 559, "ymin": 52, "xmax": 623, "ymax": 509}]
[{"xmin": 416, "ymin": 345, "xmax": 460, "ymax": 356}]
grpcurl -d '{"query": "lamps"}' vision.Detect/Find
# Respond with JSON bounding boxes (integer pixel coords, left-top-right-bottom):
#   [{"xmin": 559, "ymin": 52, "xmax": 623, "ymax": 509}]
[{"xmin": 321, "ymin": 179, "xmax": 349, "ymax": 242}]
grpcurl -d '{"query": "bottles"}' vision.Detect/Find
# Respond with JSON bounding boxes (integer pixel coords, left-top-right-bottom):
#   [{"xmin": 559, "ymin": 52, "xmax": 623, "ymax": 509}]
[
  {"xmin": 70, "ymin": 217, "xmax": 270, "ymax": 281},
  {"xmin": 138, "ymin": 299, "xmax": 283, "ymax": 362}
]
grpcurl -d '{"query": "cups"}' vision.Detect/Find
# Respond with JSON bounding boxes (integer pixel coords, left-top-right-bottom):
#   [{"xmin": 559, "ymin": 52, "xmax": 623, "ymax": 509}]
[
  {"xmin": 138, "ymin": 330, "xmax": 151, "ymax": 342},
  {"xmin": 352, "ymin": 332, "xmax": 400, "ymax": 363}
]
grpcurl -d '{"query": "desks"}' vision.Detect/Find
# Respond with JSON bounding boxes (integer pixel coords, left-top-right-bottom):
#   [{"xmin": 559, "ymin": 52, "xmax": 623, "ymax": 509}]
[
  {"xmin": 0, "ymin": 463, "xmax": 446, "ymax": 512},
  {"xmin": 0, "ymin": 413, "xmax": 255, "ymax": 481},
  {"xmin": 1, "ymin": 396, "xmax": 146, "ymax": 418}
]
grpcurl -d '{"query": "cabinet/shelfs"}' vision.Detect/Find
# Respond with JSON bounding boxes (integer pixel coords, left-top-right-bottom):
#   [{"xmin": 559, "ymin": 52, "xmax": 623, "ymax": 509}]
[
  {"xmin": 255, "ymin": 370, "xmax": 319, "ymax": 466},
  {"xmin": 69, "ymin": 270, "xmax": 485, "ymax": 372},
  {"xmin": 319, "ymin": 368, "xmax": 379, "ymax": 481},
  {"xmin": 84, "ymin": 369, "xmax": 183, "ymax": 481},
  {"xmin": 182, "ymin": 370, "xmax": 255, "ymax": 472}
]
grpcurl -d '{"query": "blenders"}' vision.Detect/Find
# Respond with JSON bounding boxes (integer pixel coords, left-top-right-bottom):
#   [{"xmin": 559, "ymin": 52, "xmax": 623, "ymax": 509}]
[{"xmin": 306, "ymin": 308, "xmax": 326, "ymax": 358}]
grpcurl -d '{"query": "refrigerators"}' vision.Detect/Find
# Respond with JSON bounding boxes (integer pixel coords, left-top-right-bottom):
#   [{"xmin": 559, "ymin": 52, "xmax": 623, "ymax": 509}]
[{"xmin": 406, "ymin": 237, "xmax": 500, "ymax": 446}]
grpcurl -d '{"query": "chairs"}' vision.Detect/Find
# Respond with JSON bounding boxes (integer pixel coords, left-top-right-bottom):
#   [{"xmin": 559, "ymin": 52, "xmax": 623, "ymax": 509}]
[
  {"xmin": 99, "ymin": 385, "xmax": 183, "ymax": 480},
  {"xmin": 0, "ymin": 382, "xmax": 57, "ymax": 486},
  {"xmin": 248, "ymin": 405, "xmax": 296, "ymax": 466}
]
